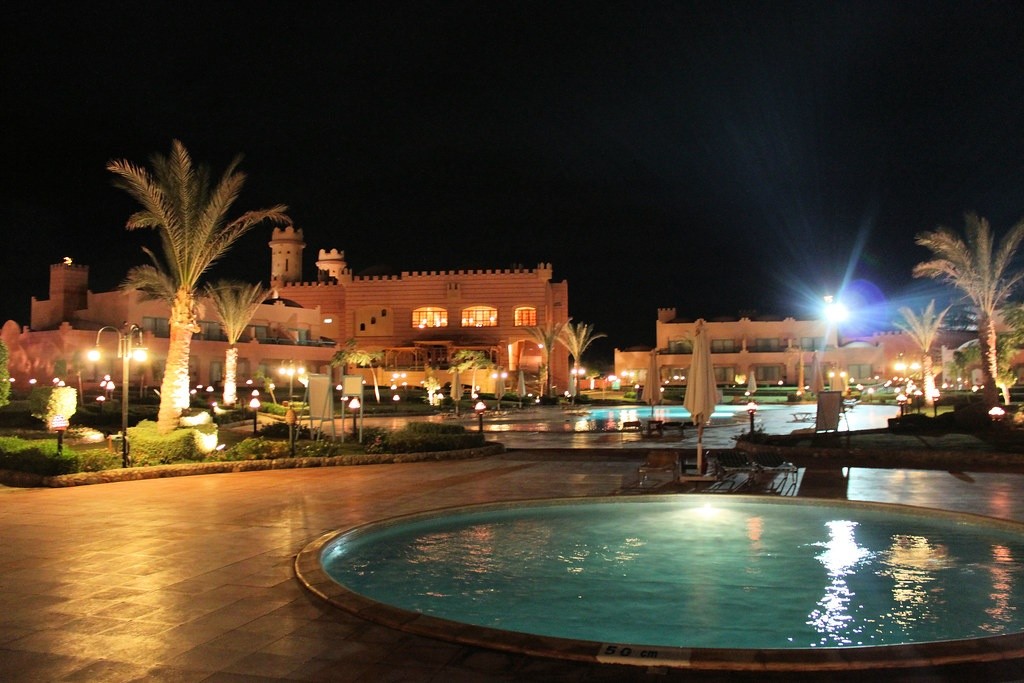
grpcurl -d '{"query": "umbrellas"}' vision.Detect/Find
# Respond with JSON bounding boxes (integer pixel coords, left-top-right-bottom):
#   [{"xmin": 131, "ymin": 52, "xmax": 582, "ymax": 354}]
[
  {"xmin": 568, "ymin": 370, "xmax": 577, "ymax": 406},
  {"xmin": 747, "ymin": 371, "xmax": 757, "ymax": 402},
  {"xmin": 494, "ymin": 366, "xmax": 506, "ymax": 411},
  {"xmin": 640, "ymin": 349, "xmax": 662, "ymax": 418},
  {"xmin": 516, "ymin": 368, "xmax": 526, "ymax": 409},
  {"xmin": 681, "ymin": 319, "xmax": 723, "ymax": 475},
  {"xmin": 450, "ymin": 368, "xmax": 464, "ymax": 416},
  {"xmin": 809, "ymin": 353, "xmax": 824, "ymax": 397}
]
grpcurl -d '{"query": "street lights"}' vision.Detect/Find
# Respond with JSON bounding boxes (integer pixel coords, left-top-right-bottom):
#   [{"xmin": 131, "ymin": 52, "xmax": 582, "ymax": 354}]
[
  {"xmin": 490, "ymin": 365, "xmax": 507, "ymax": 411},
  {"xmin": 570, "ymin": 368, "xmax": 587, "ymax": 400},
  {"xmin": 89, "ymin": 324, "xmax": 150, "ymax": 468}
]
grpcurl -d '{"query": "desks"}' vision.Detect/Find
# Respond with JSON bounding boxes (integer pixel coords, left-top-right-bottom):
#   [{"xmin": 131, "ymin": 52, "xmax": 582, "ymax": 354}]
[{"xmin": 646, "ymin": 420, "xmax": 664, "ymax": 436}]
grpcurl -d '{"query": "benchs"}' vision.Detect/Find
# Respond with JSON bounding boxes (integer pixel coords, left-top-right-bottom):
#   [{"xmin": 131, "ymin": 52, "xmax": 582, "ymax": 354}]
[{"xmin": 790, "ymin": 412, "xmax": 812, "ymax": 421}]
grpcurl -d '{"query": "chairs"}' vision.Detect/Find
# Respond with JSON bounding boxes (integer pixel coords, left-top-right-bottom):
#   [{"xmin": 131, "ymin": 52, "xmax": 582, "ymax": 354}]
[
  {"xmin": 716, "ymin": 451, "xmax": 759, "ymax": 487},
  {"xmin": 841, "ymin": 400, "xmax": 861, "ymax": 409},
  {"xmin": 750, "ymin": 448, "xmax": 796, "ymax": 482},
  {"xmin": 639, "ymin": 451, "xmax": 680, "ymax": 488},
  {"xmin": 622, "ymin": 421, "xmax": 642, "ymax": 434}
]
